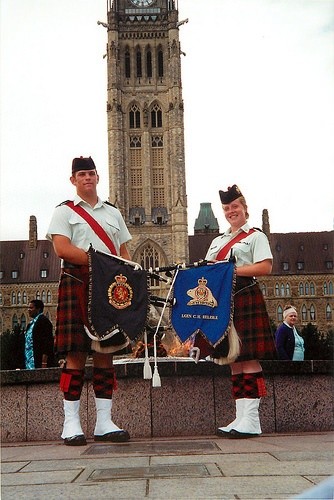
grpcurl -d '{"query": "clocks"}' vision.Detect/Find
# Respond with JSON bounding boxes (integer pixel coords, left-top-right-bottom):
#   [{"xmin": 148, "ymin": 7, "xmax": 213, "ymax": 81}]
[{"xmin": 127, "ymin": 0, "xmax": 157, "ymax": 8}]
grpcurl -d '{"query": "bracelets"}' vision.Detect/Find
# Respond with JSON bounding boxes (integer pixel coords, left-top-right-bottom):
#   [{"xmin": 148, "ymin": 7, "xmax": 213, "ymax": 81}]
[{"xmin": 42, "ymin": 363, "xmax": 47, "ymax": 364}]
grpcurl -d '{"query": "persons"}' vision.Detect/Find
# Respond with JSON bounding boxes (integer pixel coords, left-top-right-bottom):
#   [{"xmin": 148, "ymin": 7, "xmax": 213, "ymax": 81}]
[
  {"xmin": 45, "ymin": 158, "xmax": 132, "ymax": 446},
  {"xmin": 274, "ymin": 304, "xmax": 304, "ymax": 361},
  {"xmin": 24, "ymin": 300, "xmax": 54, "ymax": 369},
  {"xmin": 205, "ymin": 184, "xmax": 274, "ymax": 439}
]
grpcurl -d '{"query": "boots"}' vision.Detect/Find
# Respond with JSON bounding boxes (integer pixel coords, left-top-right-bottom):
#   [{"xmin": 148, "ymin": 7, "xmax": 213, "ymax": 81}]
[
  {"xmin": 94, "ymin": 397, "xmax": 130, "ymax": 442},
  {"xmin": 60, "ymin": 399, "xmax": 87, "ymax": 446},
  {"xmin": 229, "ymin": 398, "xmax": 262, "ymax": 438},
  {"xmin": 218, "ymin": 398, "xmax": 244, "ymax": 436}
]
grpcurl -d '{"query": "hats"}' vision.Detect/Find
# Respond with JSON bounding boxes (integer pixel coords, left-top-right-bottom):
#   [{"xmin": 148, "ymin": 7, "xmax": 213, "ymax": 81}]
[
  {"xmin": 282, "ymin": 305, "xmax": 296, "ymax": 319},
  {"xmin": 72, "ymin": 157, "xmax": 96, "ymax": 173},
  {"xmin": 219, "ymin": 184, "xmax": 242, "ymax": 204}
]
